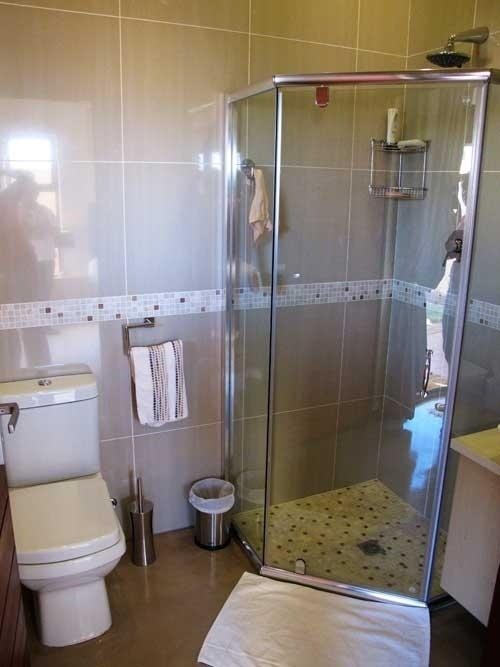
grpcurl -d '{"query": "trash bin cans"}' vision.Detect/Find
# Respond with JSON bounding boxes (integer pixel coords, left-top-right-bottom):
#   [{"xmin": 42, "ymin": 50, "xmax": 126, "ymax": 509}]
[{"xmin": 188, "ymin": 478, "xmax": 236, "ymax": 551}]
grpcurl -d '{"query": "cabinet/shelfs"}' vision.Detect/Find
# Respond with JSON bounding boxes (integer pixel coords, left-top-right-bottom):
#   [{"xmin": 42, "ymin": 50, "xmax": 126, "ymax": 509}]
[{"xmin": 364, "ymin": 134, "xmax": 429, "ymax": 200}]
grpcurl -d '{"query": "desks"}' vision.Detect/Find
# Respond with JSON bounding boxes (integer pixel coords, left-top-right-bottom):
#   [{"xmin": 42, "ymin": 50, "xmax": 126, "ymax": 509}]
[{"xmin": 440, "ymin": 426, "xmax": 500, "ymax": 628}]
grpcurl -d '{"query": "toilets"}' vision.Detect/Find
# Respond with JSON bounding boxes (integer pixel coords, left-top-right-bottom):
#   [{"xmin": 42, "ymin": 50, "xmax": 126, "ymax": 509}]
[{"xmin": 0, "ymin": 372, "xmax": 127, "ymax": 649}]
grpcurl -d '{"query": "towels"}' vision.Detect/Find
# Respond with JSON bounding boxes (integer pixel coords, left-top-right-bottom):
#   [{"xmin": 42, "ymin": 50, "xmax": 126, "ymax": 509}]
[
  {"xmin": 129, "ymin": 338, "xmax": 190, "ymax": 428},
  {"xmin": 193, "ymin": 567, "xmax": 434, "ymax": 667},
  {"xmin": 245, "ymin": 167, "xmax": 274, "ymax": 244}
]
